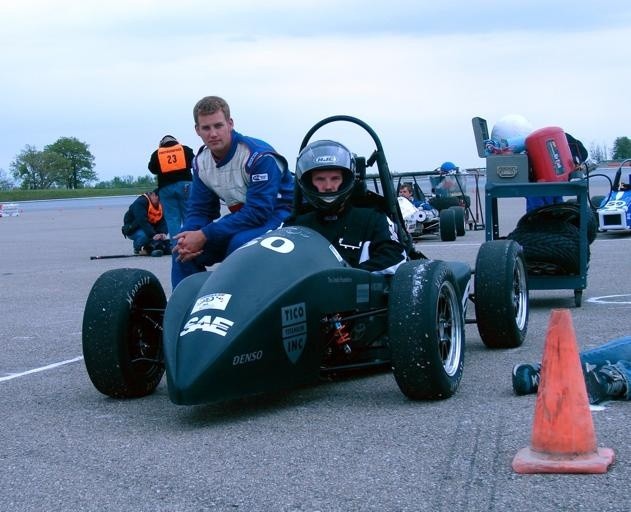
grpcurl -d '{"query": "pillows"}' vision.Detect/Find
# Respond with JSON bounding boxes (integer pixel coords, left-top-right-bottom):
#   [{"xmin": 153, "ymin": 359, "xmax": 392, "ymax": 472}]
[{"xmin": 296, "ymin": 139, "xmax": 357, "ymax": 209}]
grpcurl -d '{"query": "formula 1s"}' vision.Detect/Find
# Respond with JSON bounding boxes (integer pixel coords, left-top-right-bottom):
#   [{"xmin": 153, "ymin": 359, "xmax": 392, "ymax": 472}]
[
  {"xmin": 395, "ymin": 175, "xmax": 466, "ymax": 241},
  {"xmin": 82, "ymin": 115, "xmax": 530, "ymax": 403}
]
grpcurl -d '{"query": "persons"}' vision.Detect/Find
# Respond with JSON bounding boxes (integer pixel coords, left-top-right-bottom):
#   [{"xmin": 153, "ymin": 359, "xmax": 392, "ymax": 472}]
[
  {"xmin": 122, "ymin": 188, "xmax": 169, "ymax": 257},
  {"xmin": 399, "ymin": 183, "xmax": 435, "ymax": 211},
  {"xmin": 170, "ymin": 96, "xmax": 299, "ymax": 293},
  {"xmin": 511, "ymin": 333, "xmax": 631, "ymax": 406},
  {"xmin": 148, "ymin": 135, "xmax": 196, "ymax": 251},
  {"xmin": 281, "ymin": 138, "xmax": 409, "ymax": 278}
]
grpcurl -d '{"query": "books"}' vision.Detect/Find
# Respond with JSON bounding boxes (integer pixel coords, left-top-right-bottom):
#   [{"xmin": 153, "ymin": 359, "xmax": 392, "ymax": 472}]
[{"xmin": 471, "ymin": 117, "xmax": 530, "ymax": 185}]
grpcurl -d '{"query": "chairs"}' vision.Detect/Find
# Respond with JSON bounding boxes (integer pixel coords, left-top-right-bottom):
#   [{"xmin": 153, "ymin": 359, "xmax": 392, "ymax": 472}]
[
  {"xmin": 512, "ymin": 361, "xmax": 541, "ymax": 395},
  {"xmin": 582, "ymin": 360, "xmax": 613, "ymax": 405}
]
